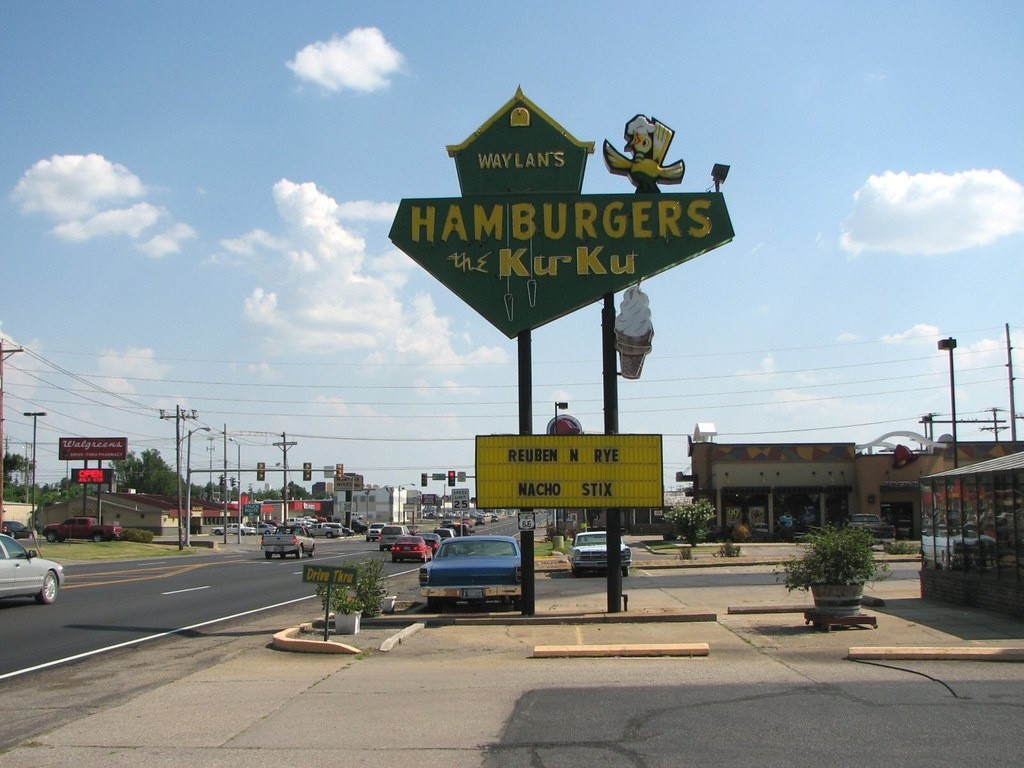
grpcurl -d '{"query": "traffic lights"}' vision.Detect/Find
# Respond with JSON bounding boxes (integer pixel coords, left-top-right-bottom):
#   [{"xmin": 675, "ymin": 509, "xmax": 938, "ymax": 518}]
[{"xmin": 448, "ymin": 471, "xmax": 456, "ymax": 486}]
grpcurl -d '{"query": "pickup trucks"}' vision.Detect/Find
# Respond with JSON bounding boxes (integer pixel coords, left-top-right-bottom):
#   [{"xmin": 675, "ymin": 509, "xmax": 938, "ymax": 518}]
[
  {"xmin": 261, "ymin": 526, "xmax": 316, "ymax": 560},
  {"xmin": 307, "ymin": 524, "xmax": 343, "ymax": 539},
  {"xmin": 850, "ymin": 513, "xmax": 897, "ymax": 539},
  {"xmin": 42, "ymin": 515, "xmax": 123, "ymax": 542},
  {"xmin": 366, "ymin": 523, "xmax": 388, "ymax": 542}
]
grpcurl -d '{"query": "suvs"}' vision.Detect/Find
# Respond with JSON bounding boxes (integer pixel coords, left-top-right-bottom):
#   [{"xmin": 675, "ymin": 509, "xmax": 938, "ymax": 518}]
[{"xmin": 379, "ymin": 525, "xmax": 410, "ymax": 552}]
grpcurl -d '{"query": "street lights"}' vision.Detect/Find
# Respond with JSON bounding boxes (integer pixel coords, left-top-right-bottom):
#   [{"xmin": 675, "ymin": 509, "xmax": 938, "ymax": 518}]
[
  {"xmin": 23, "ymin": 411, "xmax": 48, "ymax": 539},
  {"xmin": 552, "ymin": 402, "xmax": 570, "ymax": 548},
  {"xmin": 398, "ymin": 483, "xmax": 416, "ymax": 524},
  {"xmin": 228, "ymin": 437, "xmax": 243, "ymax": 545},
  {"xmin": 177, "ymin": 425, "xmax": 212, "ymax": 549},
  {"xmin": 936, "ymin": 337, "xmax": 959, "ymax": 472}
]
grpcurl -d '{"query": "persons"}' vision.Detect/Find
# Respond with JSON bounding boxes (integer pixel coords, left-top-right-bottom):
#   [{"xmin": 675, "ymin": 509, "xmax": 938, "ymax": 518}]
[{"xmin": 288, "ymin": 529, "xmax": 295, "ymax": 534}]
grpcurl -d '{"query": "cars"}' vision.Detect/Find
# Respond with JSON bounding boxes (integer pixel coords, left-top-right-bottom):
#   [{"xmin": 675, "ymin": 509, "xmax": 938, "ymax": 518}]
[
  {"xmin": 1, "ymin": 521, "xmax": 33, "ymax": 540},
  {"xmin": 407, "ymin": 511, "xmax": 499, "ymax": 540},
  {"xmin": 894, "ymin": 520, "xmax": 914, "ymax": 539},
  {"xmin": 391, "ymin": 536, "xmax": 434, "ymax": 562},
  {"xmin": 210, "ymin": 522, "xmax": 257, "ymax": 536},
  {"xmin": 0, "ymin": 534, "xmax": 65, "ymax": 605},
  {"xmin": 753, "ymin": 523, "xmax": 768, "ymax": 533},
  {"xmin": 244, "ymin": 515, "xmax": 367, "ymax": 538},
  {"xmin": 509, "ymin": 513, "xmax": 515, "ymax": 519},
  {"xmin": 418, "ymin": 535, "xmax": 522, "ymax": 611},
  {"xmin": 416, "ymin": 533, "xmax": 445, "ymax": 555},
  {"xmin": 923, "ymin": 525, "xmax": 997, "ymax": 569},
  {"xmin": 568, "ymin": 531, "xmax": 632, "ymax": 577}
]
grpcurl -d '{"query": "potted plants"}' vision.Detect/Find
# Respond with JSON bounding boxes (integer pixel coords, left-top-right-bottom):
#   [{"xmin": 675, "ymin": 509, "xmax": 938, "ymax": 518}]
[
  {"xmin": 773, "ymin": 525, "xmax": 894, "ymax": 615},
  {"xmin": 315, "ymin": 554, "xmax": 397, "ymax": 634}
]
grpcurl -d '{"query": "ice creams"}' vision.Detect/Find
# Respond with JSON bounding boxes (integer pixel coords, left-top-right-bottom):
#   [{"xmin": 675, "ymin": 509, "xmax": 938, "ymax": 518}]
[{"xmin": 614, "ymin": 281, "xmax": 654, "ymax": 380}]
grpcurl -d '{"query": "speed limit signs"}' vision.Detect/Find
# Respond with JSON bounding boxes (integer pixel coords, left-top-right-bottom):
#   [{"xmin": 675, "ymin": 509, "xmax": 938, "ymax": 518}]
[{"xmin": 451, "ymin": 488, "xmax": 470, "ymax": 511}]
